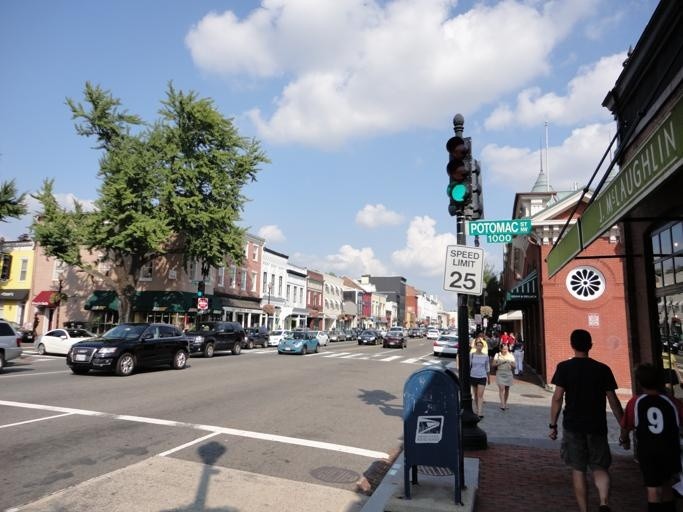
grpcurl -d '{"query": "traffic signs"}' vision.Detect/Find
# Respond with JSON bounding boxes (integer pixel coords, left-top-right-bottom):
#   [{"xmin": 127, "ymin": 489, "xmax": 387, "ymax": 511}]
[
  {"xmin": 442, "ymin": 244, "xmax": 484, "ymax": 297},
  {"xmin": 466, "ymin": 220, "xmax": 531, "ymax": 245}
]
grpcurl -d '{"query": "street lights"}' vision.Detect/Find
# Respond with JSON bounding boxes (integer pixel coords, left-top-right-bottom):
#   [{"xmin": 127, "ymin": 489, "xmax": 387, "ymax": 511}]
[{"xmin": 266, "ymin": 282, "xmax": 272, "ymax": 330}]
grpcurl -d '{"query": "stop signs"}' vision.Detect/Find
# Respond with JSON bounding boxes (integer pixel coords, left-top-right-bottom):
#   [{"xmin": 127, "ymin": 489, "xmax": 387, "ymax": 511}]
[{"xmin": 197, "ymin": 298, "xmax": 208, "ymax": 309}]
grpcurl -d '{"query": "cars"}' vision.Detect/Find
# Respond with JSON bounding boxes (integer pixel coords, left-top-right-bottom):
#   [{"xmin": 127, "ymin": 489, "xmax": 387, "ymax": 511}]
[
  {"xmin": 433, "ymin": 334, "xmax": 458, "ymax": 356},
  {"xmin": 65, "ymin": 322, "xmax": 189, "ymax": 375},
  {"xmin": 34, "ymin": 328, "xmax": 97, "ymax": 354},
  {"xmin": 243, "ymin": 328, "xmax": 268, "ymax": 349},
  {"xmin": 7, "ymin": 320, "xmax": 34, "ymax": 343},
  {"xmin": 268, "ymin": 326, "xmax": 440, "ymax": 356},
  {"xmin": 0, "ymin": 320, "xmax": 21, "ymax": 369}
]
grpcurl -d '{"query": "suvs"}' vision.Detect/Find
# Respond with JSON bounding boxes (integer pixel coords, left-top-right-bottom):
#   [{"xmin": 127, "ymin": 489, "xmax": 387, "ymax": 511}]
[{"xmin": 185, "ymin": 321, "xmax": 246, "ymax": 358}]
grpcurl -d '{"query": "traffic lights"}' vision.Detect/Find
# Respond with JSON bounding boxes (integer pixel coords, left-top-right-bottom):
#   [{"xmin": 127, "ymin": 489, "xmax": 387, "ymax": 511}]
[
  {"xmin": 196, "ymin": 280, "xmax": 205, "ymax": 298},
  {"xmin": 445, "ymin": 136, "xmax": 472, "ymax": 214}
]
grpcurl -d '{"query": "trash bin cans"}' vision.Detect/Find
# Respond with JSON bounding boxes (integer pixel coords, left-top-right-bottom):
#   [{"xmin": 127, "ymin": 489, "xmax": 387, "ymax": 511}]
[{"xmin": 402, "ymin": 365, "xmax": 464, "ymax": 502}]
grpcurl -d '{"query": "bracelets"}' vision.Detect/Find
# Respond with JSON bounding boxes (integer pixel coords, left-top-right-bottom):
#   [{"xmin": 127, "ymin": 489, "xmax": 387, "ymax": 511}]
[
  {"xmin": 619, "ymin": 438, "xmax": 630, "ymax": 445},
  {"xmin": 549, "ymin": 423, "xmax": 559, "ymax": 428}
]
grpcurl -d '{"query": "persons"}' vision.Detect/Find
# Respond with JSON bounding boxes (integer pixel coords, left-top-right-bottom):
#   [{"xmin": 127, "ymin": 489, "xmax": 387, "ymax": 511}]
[
  {"xmin": 620, "ymin": 362, "xmax": 681, "ymax": 511},
  {"xmin": 469, "ymin": 323, "xmax": 525, "ymax": 419},
  {"xmin": 547, "ymin": 329, "xmax": 623, "ymax": 510}
]
